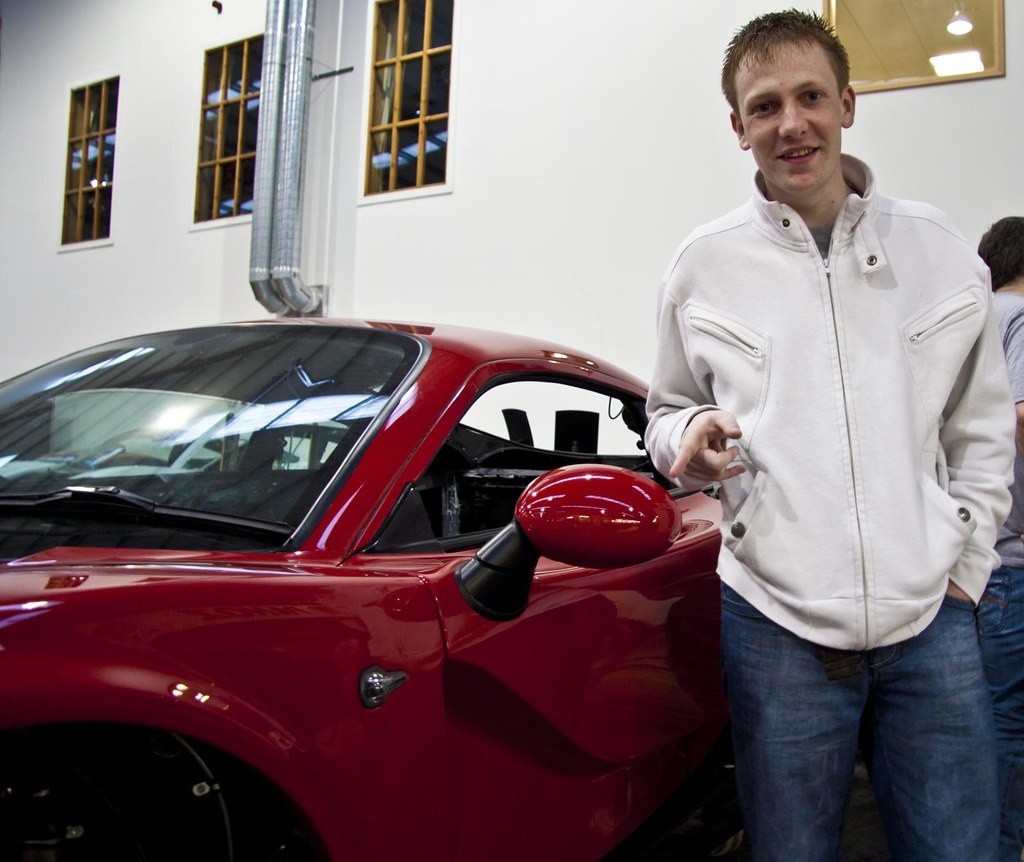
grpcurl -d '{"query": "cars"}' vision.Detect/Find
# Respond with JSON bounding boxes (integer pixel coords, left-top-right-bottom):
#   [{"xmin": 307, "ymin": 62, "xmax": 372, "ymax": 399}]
[{"xmin": 0, "ymin": 320, "xmax": 740, "ymax": 862}]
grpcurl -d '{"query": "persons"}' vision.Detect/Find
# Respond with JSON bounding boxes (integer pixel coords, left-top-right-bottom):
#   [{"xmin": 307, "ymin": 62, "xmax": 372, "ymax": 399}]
[{"xmin": 644, "ymin": 11, "xmax": 1024, "ymax": 862}]
